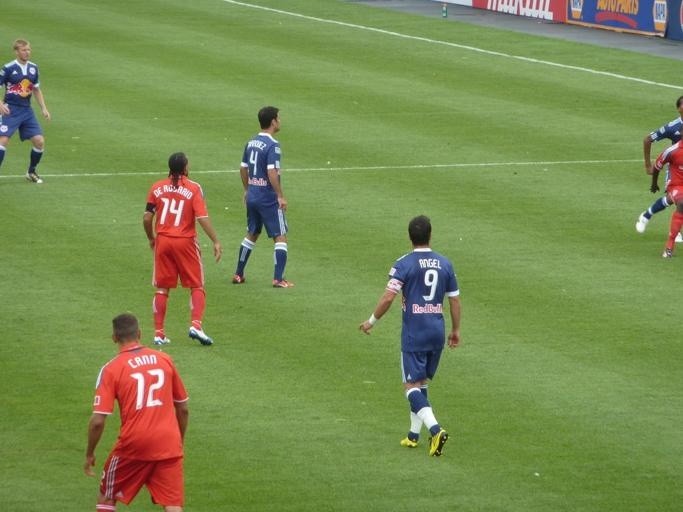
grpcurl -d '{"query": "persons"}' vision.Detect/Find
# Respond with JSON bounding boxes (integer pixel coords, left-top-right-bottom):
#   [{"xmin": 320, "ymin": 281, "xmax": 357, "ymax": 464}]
[
  {"xmin": 141, "ymin": 153, "xmax": 223, "ymax": 345},
  {"xmin": 649, "ymin": 124, "xmax": 683, "ymax": 259},
  {"xmin": 232, "ymin": 107, "xmax": 297, "ymax": 287},
  {"xmin": 357, "ymin": 215, "xmax": 462, "ymax": 457},
  {"xmin": 84, "ymin": 313, "xmax": 190, "ymax": 511},
  {"xmin": 0, "ymin": 39, "xmax": 52, "ymax": 184},
  {"xmin": 637, "ymin": 96, "xmax": 683, "ymax": 243}
]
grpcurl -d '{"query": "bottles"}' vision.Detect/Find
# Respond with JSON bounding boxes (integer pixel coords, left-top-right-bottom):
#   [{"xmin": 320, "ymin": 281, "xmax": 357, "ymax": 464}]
[{"xmin": 441, "ymin": 4, "xmax": 448, "ymax": 17}]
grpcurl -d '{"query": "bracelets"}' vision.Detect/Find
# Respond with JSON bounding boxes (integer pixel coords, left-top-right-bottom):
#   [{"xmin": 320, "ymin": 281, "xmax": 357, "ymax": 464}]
[{"xmin": 369, "ymin": 314, "xmax": 380, "ymax": 326}]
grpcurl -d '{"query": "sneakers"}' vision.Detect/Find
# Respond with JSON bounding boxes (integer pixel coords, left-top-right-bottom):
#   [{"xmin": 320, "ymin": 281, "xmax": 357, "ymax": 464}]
[
  {"xmin": 401, "ymin": 428, "xmax": 448, "ymax": 456},
  {"xmin": 26, "ymin": 171, "xmax": 42, "ymax": 183},
  {"xmin": 154, "ymin": 336, "xmax": 170, "ymax": 344},
  {"xmin": 636, "ymin": 211, "xmax": 648, "ymax": 233},
  {"xmin": 663, "ymin": 248, "xmax": 672, "ymax": 257},
  {"xmin": 233, "ymin": 275, "xmax": 244, "ymax": 283},
  {"xmin": 189, "ymin": 326, "xmax": 213, "ymax": 345},
  {"xmin": 273, "ymin": 279, "xmax": 294, "ymax": 288},
  {"xmin": 675, "ymin": 232, "xmax": 682, "ymax": 242}
]
